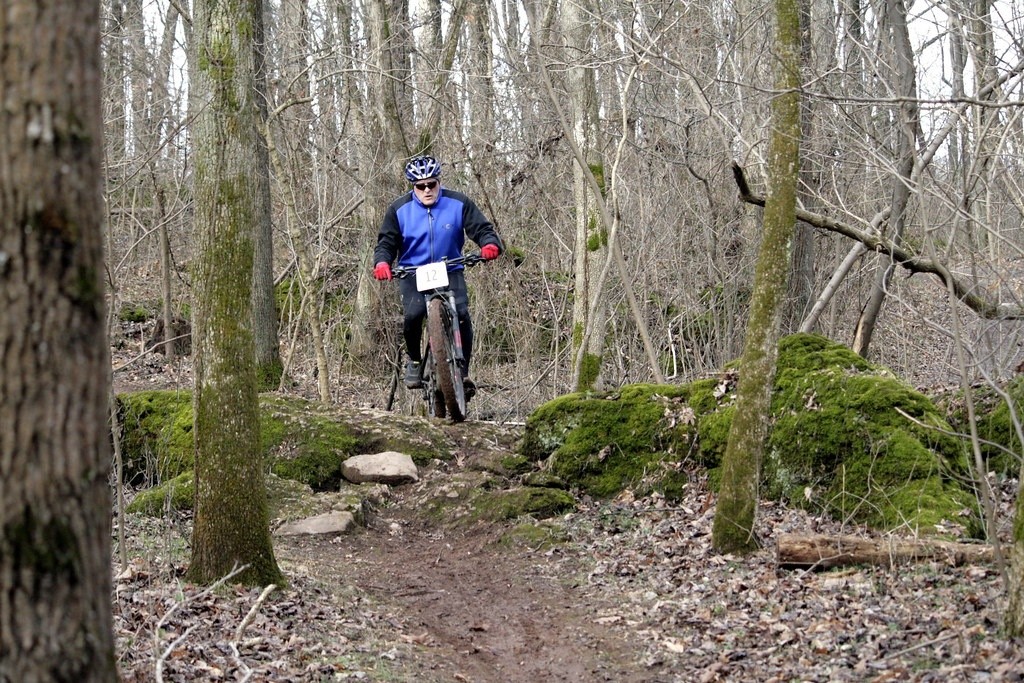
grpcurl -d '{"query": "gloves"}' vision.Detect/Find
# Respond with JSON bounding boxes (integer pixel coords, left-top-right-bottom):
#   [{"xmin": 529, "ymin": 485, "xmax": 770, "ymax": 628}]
[
  {"xmin": 373, "ymin": 261, "xmax": 392, "ymax": 282},
  {"xmin": 481, "ymin": 243, "xmax": 499, "ymax": 261}
]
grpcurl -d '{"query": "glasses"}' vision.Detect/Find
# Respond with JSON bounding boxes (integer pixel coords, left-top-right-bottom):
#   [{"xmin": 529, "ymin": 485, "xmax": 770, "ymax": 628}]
[{"xmin": 414, "ymin": 179, "xmax": 437, "ymax": 191}]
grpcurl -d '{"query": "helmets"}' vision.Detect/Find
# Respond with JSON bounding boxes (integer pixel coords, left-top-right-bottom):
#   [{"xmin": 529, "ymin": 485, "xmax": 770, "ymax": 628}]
[{"xmin": 405, "ymin": 155, "xmax": 441, "ymax": 182}]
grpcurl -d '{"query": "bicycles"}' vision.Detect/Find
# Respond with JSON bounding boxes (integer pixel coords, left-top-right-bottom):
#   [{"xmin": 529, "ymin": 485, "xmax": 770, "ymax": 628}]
[{"xmin": 372, "ymin": 247, "xmax": 500, "ymax": 420}]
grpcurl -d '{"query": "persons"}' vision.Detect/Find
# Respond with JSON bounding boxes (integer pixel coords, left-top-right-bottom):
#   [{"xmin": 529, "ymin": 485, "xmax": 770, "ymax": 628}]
[{"xmin": 372, "ymin": 155, "xmax": 501, "ymax": 402}]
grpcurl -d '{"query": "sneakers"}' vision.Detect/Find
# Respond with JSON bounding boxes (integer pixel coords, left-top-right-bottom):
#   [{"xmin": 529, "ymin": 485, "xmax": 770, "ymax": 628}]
[
  {"xmin": 461, "ymin": 376, "xmax": 476, "ymax": 402},
  {"xmin": 404, "ymin": 358, "xmax": 422, "ymax": 387}
]
